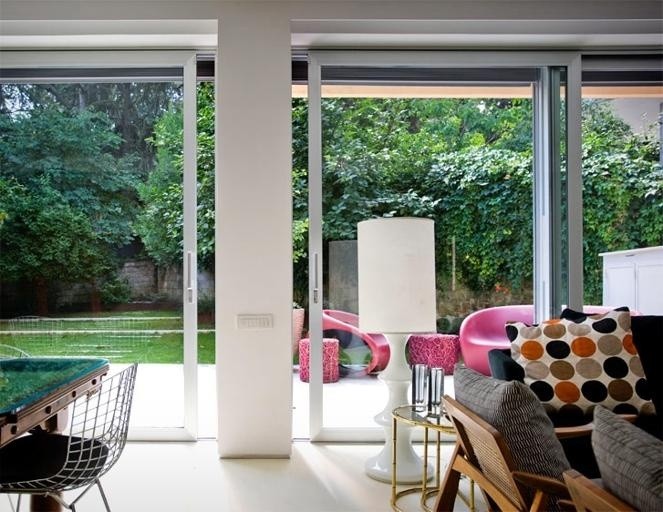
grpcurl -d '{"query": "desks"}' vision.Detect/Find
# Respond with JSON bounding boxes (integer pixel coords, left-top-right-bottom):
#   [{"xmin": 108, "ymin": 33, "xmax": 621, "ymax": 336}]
[{"xmin": 1, "ymin": 357, "xmax": 110, "ymax": 512}]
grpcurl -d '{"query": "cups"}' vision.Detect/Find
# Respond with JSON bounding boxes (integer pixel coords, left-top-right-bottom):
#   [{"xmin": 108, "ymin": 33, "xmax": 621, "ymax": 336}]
[
  {"xmin": 410, "ymin": 364, "xmax": 428, "ymax": 412},
  {"xmin": 428, "ymin": 368, "xmax": 444, "ymax": 407}
]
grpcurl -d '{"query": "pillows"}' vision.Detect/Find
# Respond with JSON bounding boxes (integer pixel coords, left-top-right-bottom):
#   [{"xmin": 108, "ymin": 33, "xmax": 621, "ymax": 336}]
[
  {"xmin": 591, "ymin": 404, "xmax": 663, "ymax": 506},
  {"xmin": 452, "ymin": 363, "xmax": 571, "ymax": 494},
  {"xmin": 504, "ymin": 306, "xmax": 656, "ymax": 421}
]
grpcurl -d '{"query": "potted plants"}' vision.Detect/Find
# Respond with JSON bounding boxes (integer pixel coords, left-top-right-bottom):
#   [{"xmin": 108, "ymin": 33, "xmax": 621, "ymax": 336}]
[{"xmin": 292, "ymin": 301, "xmax": 305, "ymax": 356}]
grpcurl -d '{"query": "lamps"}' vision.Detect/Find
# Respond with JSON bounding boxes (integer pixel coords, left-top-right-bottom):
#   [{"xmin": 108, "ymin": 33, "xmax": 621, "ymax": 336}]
[{"xmin": 356, "ymin": 217, "xmax": 439, "ymax": 486}]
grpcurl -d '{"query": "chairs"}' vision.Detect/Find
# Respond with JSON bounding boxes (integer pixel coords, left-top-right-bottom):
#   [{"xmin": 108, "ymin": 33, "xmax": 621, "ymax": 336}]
[
  {"xmin": 0, "ymin": 360, "xmax": 138, "ymax": 512},
  {"xmin": 431, "ymin": 394, "xmax": 572, "ymax": 512}
]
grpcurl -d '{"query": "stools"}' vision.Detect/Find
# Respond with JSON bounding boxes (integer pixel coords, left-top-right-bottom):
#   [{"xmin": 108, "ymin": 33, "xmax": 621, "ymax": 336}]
[
  {"xmin": 298, "ymin": 338, "xmax": 341, "ymax": 384},
  {"xmin": 407, "ymin": 333, "xmax": 463, "ymax": 376}
]
